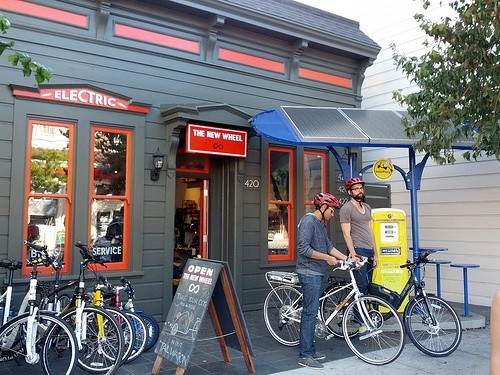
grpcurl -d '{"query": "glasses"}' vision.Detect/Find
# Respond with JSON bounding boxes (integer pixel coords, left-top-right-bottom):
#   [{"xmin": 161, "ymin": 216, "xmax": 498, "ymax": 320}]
[
  {"xmin": 351, "ymin": 187, "xmax": 364, "ymax": 192},
  {"xmin": 328, "ymin": 206, "xmax": 335, "ymax": 213}
]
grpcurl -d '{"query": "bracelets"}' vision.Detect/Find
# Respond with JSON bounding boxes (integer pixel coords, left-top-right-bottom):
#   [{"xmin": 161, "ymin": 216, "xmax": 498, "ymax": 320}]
[{"xmin": 346, "ymin": 257, "xmax": 349, "ymax": 261}]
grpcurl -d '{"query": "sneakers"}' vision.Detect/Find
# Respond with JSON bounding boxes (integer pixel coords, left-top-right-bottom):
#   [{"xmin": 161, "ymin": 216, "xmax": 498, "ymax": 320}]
[
  {"xmin": 359, "ymin": 328, "xmax": 382, "ymax": 340},
  {"xmin": 298, "ymin": 358, "xmax": 324, "ymax": 369},
  {"xmin": 312, "ymin": 352, "xmax": 326, "ymax": 360}
]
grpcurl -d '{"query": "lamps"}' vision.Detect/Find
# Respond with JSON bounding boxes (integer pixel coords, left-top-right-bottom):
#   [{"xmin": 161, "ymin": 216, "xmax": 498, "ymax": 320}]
[{"xmin": 150, "ymin": 147, "xmax": 166, "ymax": 181}]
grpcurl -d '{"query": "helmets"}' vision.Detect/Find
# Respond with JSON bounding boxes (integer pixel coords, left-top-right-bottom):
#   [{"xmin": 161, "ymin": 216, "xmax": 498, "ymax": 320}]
[
  {"xmin": 345, "ymin": 178, "xmax": 365, "ymax": 191},
  {"xmin": 314, "ymin": 192, "xmax": 340, "ymax": 209}
]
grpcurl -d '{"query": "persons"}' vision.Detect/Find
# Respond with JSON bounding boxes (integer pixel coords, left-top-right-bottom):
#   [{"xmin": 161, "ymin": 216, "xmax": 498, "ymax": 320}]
[
  {"xmin": 296, "ymin": 192, "xmax": 361, "ymax": 369},
  {"xmin": 339, "ymin": 177, "xmax": 380, "ymax": 325},
  {"xmin": 489, "ymin": 290, "xmax": 500, "ymax": 375}
]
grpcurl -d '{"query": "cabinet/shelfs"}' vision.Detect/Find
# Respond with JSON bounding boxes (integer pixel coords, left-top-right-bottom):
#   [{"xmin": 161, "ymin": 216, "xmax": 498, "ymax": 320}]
[{"xmin": 182, "ymin": 200, "xmax": 200, "ymax": 224}]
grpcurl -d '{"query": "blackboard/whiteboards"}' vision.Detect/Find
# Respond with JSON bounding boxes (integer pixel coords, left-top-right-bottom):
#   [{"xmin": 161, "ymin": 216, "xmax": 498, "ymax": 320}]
[{"xmin": 153, "ymin": 258, "xmax": 256, "ymax": 368}]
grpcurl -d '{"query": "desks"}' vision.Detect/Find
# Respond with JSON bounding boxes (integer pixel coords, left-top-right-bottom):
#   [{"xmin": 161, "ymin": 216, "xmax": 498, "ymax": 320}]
[
  {"xmin": 425, "ymin": 260, "xmax": 451, "ymax": 298},
  {"xmin": 409, "ymin": 247, "xmax": 448, "ymax": 253},
  {"xmin": 450, "ymin": 264, "xmax": 480, "ymax": 318}
]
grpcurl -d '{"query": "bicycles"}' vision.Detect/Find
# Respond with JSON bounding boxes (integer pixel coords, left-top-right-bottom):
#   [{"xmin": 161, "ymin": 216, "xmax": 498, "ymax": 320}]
[
  {"xmin": 320, "ymin": 251, "xmax": 462, "ymax": 357},
  {"xmin": 263, "ymin": 252, "xmax": 406, "ymax": 366},
  {"xmin": 0, "ymin": 241, "xmax": 160, "ymax": 375}
]
